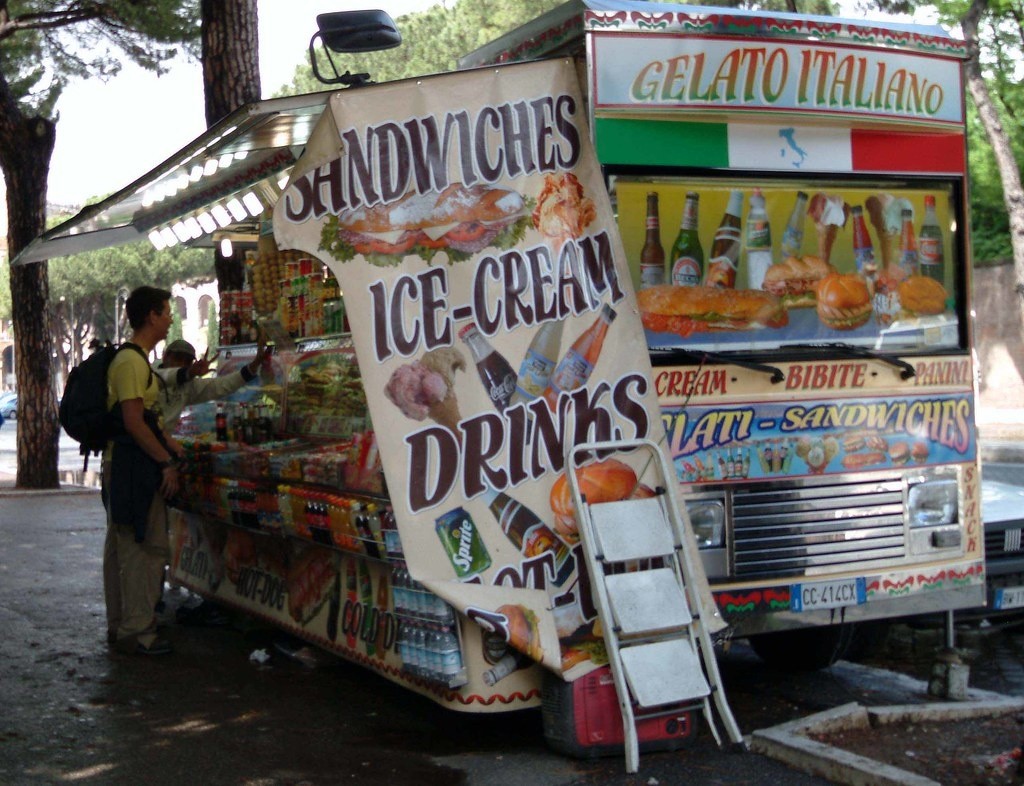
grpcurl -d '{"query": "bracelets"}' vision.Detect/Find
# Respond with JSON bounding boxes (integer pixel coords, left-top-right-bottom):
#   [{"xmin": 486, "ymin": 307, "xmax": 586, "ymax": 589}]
[{"xmin": 157, "ymin": 458, "xmax": 174, "ymax": 470}]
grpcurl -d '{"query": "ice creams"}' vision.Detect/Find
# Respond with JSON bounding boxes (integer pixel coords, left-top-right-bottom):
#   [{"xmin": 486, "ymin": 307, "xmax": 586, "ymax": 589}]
[
  {"xmin": 807, "ymin": 193, "xmax": 850, "ymax": 262},
  {"xmin": 866, "ymin": 192, "xmax": 914, "ymax": 268},
  {"xmin": 384, "ymin": 345, "xmax": 469, "ymax": 452}
]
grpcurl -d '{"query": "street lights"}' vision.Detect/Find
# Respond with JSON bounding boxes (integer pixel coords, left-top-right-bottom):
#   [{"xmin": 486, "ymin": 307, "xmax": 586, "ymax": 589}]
[
  {"xmin": 60, "ymin": 295, "xmax": 75, "ymax": 367},
  {"xmin": 114, "ymin": 287, "xmax": 131, "ymax": 350},
  {"xmin": 8, "ymin": 319, "xmax": 13, "ymax": 326}
]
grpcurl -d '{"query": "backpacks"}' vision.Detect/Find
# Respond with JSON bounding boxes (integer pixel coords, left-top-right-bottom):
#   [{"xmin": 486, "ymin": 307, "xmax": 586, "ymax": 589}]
[{"xmin": 58, "ymin": 337, "xmax": 171, "ymax": 473}]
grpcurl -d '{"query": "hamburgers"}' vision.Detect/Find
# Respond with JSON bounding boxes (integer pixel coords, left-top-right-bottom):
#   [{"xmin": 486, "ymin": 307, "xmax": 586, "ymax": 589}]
[
  {"xmin": 898, "ymin": 276, "xmax": 948, "ymax": 317},
  {"xmin": 316, "ymin": 182, "xmax": 540, "ymax": 266},
  {"xmin": 287, "ymin": 361, "xmax": 366, "ymax": 422},
  {"xmin": 818, "ymin": 273, "xmax": 872, "ymax": 331},
  {"xmin": 761, "ymin": 255, "xmax": 838, "ymax": 309},
  {"xmin": 637, "ymin": 285, "xmax": 788, "ymax": 337},
  {"xmin": 495, "ymin": 604, "xmax": 543, "ymax": 662},
  {"xmin": 550, "ymin": 459, "xmax": 654, "ymax": 543},
  {"xmin": 841, "ymin": 436, "xmax": 928, "ymax": 469}
]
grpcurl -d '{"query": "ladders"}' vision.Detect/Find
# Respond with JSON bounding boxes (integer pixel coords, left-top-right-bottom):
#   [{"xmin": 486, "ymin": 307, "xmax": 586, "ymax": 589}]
[{"xmin": 565, "ymin": 439, "xmax": 745, "ymax": 775}]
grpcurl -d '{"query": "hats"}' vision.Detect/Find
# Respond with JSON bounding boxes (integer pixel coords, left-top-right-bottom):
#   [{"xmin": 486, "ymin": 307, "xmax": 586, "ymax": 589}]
[{"xmin": 166, "ymin": 339, "xmax": 197, "ymax": 359}]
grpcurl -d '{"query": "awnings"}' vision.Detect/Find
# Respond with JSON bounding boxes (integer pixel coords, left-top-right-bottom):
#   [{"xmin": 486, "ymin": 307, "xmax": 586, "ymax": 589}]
[{"xmin": 8, "ymin": 58, "xmax": 563, "ymax": 273}]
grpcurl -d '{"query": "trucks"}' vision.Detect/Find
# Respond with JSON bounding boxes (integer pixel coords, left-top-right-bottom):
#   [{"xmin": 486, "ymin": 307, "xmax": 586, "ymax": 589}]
[{"xmin": 10, "ymin": 0, "xmax": 987, "ymax": 713}]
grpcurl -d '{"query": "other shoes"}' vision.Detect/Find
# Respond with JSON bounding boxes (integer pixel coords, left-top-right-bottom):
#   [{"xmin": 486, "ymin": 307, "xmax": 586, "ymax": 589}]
[
  {"xmin": 106, "ymin": 633, "xmax": 116, "ymax": 644},
  {"xmin": 120, "ymin": 635, "xmax": 171, "ymax": 654}
]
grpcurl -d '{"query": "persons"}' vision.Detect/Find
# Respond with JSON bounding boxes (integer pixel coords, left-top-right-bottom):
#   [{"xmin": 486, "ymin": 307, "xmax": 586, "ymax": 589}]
[
  {"xmin": 99, "ymin": 286, "xmax": 185, "ymax": 653},
  {"xmin": 153, "ymin": 337, "xmax": 266, "ymax": 433}
]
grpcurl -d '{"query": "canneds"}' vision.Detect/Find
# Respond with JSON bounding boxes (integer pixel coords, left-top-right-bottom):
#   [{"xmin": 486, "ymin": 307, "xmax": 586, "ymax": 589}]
[{"xmin": 435, "ymin": 505, "xmax": 492, "ymax": 579}]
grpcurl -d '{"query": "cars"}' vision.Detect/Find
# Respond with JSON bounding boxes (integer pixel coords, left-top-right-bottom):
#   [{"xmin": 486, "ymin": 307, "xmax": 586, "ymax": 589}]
[
  {"xmin": 0, "ymin": 392, "xmax": 62, "ymax": 420},
  {"xmin": 903, "ymin": 478, "xmax": 1024, "ymax": 628}
]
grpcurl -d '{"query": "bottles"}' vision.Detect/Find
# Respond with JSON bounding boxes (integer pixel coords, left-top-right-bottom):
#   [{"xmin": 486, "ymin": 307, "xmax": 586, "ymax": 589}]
[
  {"xmin": 230, "ymin": 304, "xmax": 241, "ymax": 345},
  {"xmin": 233, "ymin": 402, "xmax": 274, "ymax": 445},
  {"xmin": 746, "ymin": 196, "xmax": 773, "ymax": 290},
  {"xmin": 327, "ymin": 557, "xmax": 388, "ymax": 659},
  {"xmin": 682, "ymin": 438, "xmax": 793, "ymax": 483},
  {"xmin": 780, "ymin": 191, "xmax": 808, "ymax": 263},
  {"xmin": 392, "ymin": 563, "xmax": 452, "ymax": 621},
  {"xmin": 639, "ymin": 191, "xmax": 665, "ymax": 290},
  {"xmin": 478, "ymin": 477, "xmax": 575, "ymax": 587},
  {"xmin": 706, "ymin": 191, "xmax": 744, "ymax": 290},
  {"xmin": 249, "ymin": 309, "xmax": 260, "ymax": 341},
  {"xmin": 851, "ymin": 205, "xmax": 878, "ymax": 280},
  {"xmin": 175, "ymin": 472, "xmax": 405, "ymax": 560},
  {"xmin": 919, "ymin": 196, "xmax": 945, "ymax": 286},
  {"xmin": 458, "ymin": 303, "xmax": 616, "ymax": 444},
  {"xmin": 898, "ymin": 209, "xmax": 920, "ymax": 281},
  {"xmin": 481, "ymin": 644, "xmax": 535, "ymax": 686},
  {"xmin": 399, "ymin": 619, "xmax": 461, "ymax": 682},
  {"xmin": 216, "ymin": 403, "xmax": 228, "ymax": 441},
  {"xmin": 670, "ymin": 191, "xmax": 704, "ymax": 286}
]
